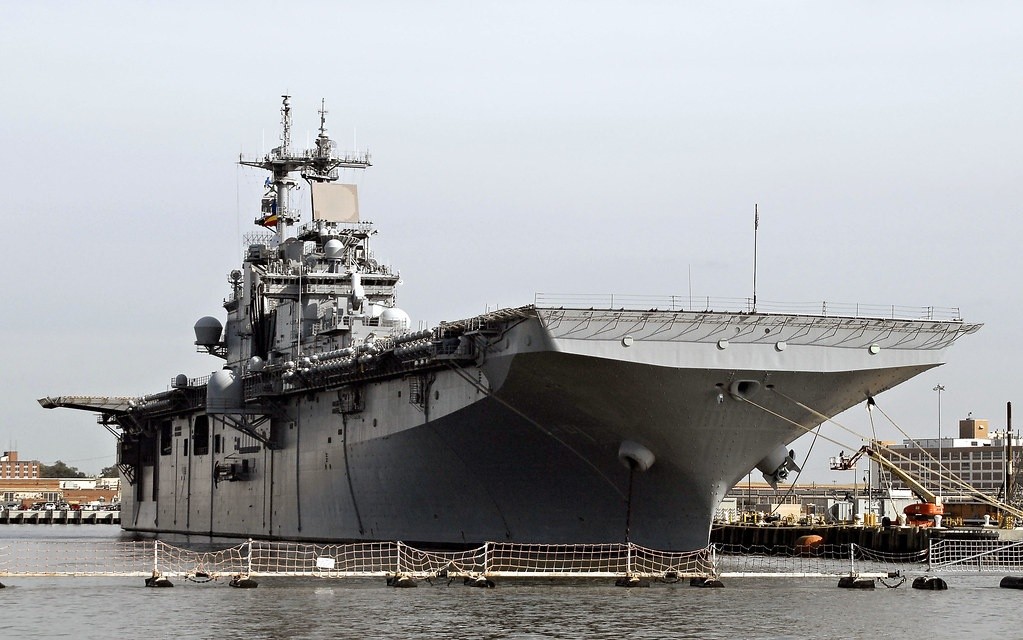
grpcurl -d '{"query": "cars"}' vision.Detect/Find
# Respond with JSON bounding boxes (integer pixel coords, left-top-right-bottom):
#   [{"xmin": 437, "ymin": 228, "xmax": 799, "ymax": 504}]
[{"xmin": 0, "ymin": 500, "xmax": 121, "ymax": 511}]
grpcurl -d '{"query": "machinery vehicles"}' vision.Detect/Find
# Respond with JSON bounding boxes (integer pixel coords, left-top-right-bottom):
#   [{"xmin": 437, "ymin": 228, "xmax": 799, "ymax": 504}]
[{"xmin": 834, "ymin": 447, "xmax": 1007, "ymax": 527}]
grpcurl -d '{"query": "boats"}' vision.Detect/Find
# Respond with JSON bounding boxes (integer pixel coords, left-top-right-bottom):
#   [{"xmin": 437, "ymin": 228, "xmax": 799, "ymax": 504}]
[{"xmin": 32, "ymin": 88, "xmax": 987, "ymax": 588}]
